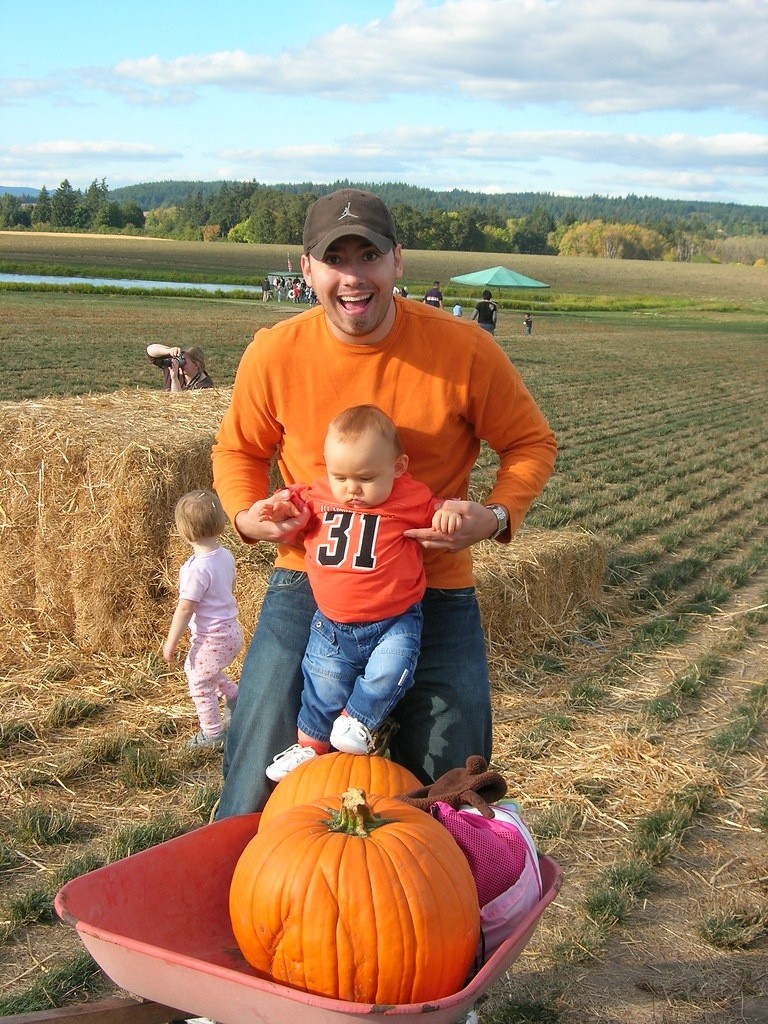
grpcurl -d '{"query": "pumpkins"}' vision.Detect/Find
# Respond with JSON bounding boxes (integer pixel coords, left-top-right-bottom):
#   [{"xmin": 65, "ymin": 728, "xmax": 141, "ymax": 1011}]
[
  {"xmin": 229, "ymin": 787, "xmax": 480, "ymax": 1006},
  {"xmin": 257, "ymin": 720, "xmax": 428, "ymax": 834}
]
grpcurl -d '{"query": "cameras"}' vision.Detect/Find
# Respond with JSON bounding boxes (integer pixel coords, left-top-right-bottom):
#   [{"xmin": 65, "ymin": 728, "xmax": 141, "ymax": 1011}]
[{"xmin": 159, "ymin": 354, "xmax": 186, "ymax": 368}]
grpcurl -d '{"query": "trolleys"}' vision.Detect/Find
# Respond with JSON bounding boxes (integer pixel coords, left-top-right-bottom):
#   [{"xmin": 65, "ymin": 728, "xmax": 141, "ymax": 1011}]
[{"xmin": 0, "ymin": 812, "xmax": 564, "ymax": 1024}]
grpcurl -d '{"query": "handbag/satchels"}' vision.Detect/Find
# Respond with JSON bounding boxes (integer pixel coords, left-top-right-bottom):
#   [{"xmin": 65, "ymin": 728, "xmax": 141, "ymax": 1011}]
[{"xmin": 428, "ymin": 798, "xmax": 543, "ymax": 981}]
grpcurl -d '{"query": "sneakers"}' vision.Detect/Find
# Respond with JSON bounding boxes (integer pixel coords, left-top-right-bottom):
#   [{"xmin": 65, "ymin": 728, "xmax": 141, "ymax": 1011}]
[
  {"xmin": 330, "ymin": 715, "xmax": 375, "ymax": 755},
  {"xmin": 266, "ymin": 743, "xmax": 319, "ymax": 782},
  {"xmin": 185, "ymin": 730, "xmax": 225, "ymax": 750},
  {"xmin": 222, "ymin": 698, "xmax": 237, "ymax": 720}
]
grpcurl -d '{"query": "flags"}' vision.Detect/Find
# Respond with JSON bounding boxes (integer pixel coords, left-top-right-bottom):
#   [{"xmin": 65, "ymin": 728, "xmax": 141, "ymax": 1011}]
[{"xmin": 288, "ymin": 255, "xmax": 292, "ymax": 268}]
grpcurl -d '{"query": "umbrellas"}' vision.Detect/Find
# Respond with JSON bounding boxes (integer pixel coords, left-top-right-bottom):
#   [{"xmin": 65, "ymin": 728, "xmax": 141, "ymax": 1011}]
[{"xmin": 450, "ymin": 267, "xmax": 551, "ymax": 291}]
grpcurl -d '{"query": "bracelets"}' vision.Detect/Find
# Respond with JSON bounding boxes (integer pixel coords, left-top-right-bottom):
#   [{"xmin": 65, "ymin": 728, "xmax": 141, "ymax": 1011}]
[{"xmin": 485, "ymin": 505, "xmax": 507, "ymax": 539}]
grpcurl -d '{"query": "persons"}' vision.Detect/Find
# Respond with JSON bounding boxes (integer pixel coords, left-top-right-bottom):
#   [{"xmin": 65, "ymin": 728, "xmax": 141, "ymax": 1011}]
[
  {"xmin": 422, "ymin": 281, "xmax": 444, "ymax": 310},
  {"xmin": 259, "ymin": 405, "xmax": 462, "ymax": 782},
  {"xmin": 523, "ymin": 313, "xmax": 533, "ymax": 337},
  {"xmin": 262, "ymin": 278, "xmax": 270, "ymax": 301},
  {"xmin": 211, "ymin": 190, "xmax": 557, "ymax": 822},
  {"xmin": 452, "ymin": 302, "xmax": 463, "ymax": 317},
  {"xmin": 274, "ymin": 276, "xmax": 321, "ymax": 307},
  {"xmin": 147, "ymin": 342, "xmax": 213, "ymax": 390},
  {"xmin": 163, "ymin": 491, "xmax": 243, "ymax": 753},
  {"xmin": 392, "ymin": 286, "xmax": 408, "ymax": 297},
  {"xmin": 471, "ymin": 290, "xmax": 497, "ymax": 334}
]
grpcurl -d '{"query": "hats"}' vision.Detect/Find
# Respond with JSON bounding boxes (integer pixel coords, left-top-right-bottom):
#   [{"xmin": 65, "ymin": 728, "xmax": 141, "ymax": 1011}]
[
  {"xmin": 303, "ymin": 187, "xmax": 398, "ymax": 260},
  {"xmin": 397, "ymin": 755, "xmax": 507, "ymax": 819}
]
